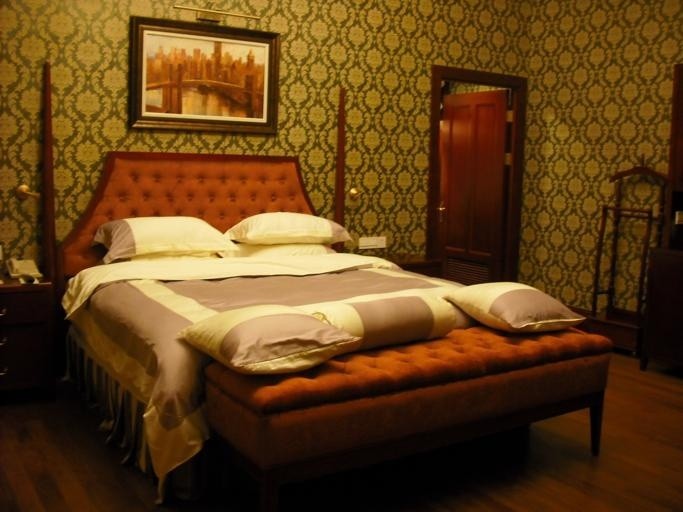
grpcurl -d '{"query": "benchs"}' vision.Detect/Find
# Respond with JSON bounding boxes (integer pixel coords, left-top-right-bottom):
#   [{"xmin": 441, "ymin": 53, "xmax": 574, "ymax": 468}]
[{"xmin": 200, "ymin": 325, "xmax": 614, "ymax": 509}]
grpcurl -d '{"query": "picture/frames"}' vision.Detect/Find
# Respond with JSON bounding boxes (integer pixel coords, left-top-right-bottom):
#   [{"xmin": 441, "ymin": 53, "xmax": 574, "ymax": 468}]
[{"xmin": 126, "ymin": 12, "xmax": 282, "ymax": 137}]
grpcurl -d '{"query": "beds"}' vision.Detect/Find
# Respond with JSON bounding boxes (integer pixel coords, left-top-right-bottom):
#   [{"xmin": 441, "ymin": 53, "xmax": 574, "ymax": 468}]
[{"xmin": 51, "ymin": 149, "xmax": 477, "ymax": 494}]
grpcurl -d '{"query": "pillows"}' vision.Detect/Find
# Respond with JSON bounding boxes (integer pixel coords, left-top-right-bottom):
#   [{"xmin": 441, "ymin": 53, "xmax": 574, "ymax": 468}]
[{"xmin": 96, "ymin": 210, "xmax": 356, "ymax": 266}]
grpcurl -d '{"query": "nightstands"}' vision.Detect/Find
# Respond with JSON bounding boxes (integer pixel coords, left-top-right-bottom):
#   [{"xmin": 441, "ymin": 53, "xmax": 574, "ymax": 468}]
[{"xmin": 1, "ymin": 277, "xmax": 59, "ymax": 402}]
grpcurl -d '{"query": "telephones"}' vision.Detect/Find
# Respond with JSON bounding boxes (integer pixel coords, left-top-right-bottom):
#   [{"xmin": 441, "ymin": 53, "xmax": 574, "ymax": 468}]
[{"xmin": 6, "ymin": 258, "xmax": 42, "ymax": 279}]
